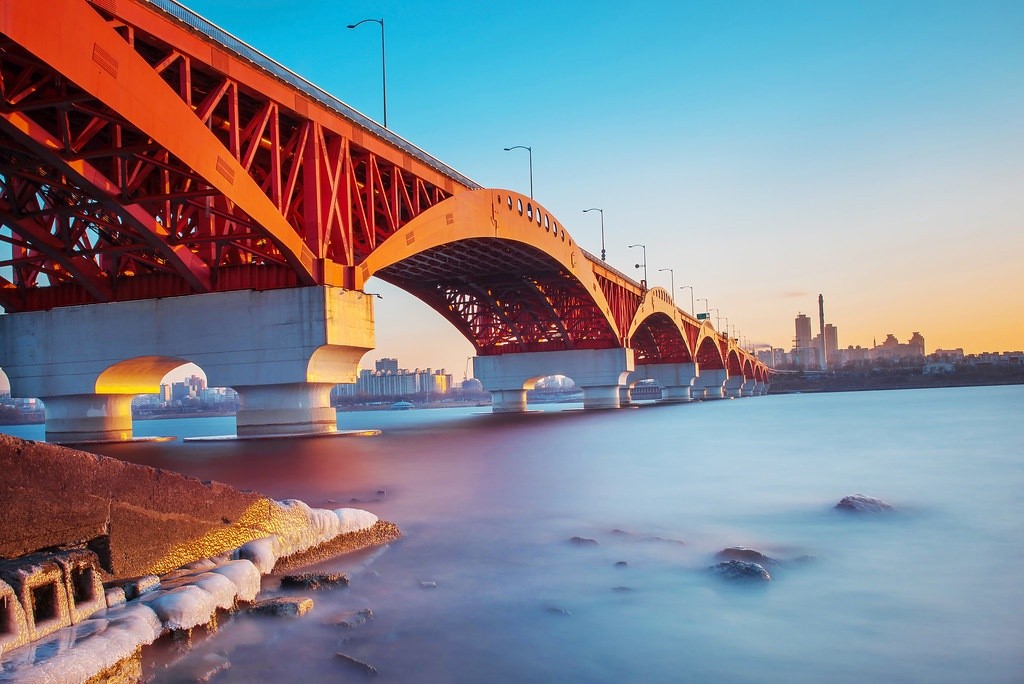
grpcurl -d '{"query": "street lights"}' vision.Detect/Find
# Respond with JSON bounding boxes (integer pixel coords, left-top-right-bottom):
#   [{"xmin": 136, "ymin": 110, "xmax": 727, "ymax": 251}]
[
  {"xmin": 346, "ymin": 19, "xmax": 388, "ymax": 130},
  {"xmin": 503, "ymin": 146, "xmax": 533, "ymax": 199},
  {"xmin": 681, "ymin": 286, "xmax": 694, "ymax": 318},
  {"xmin": 744, "ymin": 340, "xmax": 750, "ymax": 354},
  {"xmin": 733, "ymin": 331, "xmax": 740, "ymax": 347},
  {"xmin": 709, "ymin": 309, "xmax": 719, "ymax": 333},
  {"xmin": 697, "ymin": 299, "xmax": 708, "ymax": 320},
  {"xmin": 727, "ymin": 325, "xmax": 735, "ymax": 342},
  {"xmin": 719, "ymin": 317, "xmax": 728, "ymax": 339},
  {"xmin": 583, "ymin": 208, "xmax": 606, "ymax": 262},
  {"xmin": 658, "ymin": 269, "xmax": 674, "ymax": 302},
  {"xmin": 739, "ymin": 336, "xmax": 746, "ymax": 351},
  {"xmin": 749, "ymin": 343, "xmax": 754, "ymax": 355},
  {"xmin": 629, "ymin": 245, "xmax": 647, "ymax": 290}
]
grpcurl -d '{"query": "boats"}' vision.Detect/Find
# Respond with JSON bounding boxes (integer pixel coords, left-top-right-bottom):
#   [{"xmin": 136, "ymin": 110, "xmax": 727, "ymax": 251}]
[{"xmin": 390, "ymin": 402, "xmax": 415, "ymax": 411}]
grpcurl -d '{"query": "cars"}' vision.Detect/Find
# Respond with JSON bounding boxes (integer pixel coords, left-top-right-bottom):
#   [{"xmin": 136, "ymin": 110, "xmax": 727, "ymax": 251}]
[{"xmin": 749, "ymin": 351, "xmax": 755, "ymax": 355}]
